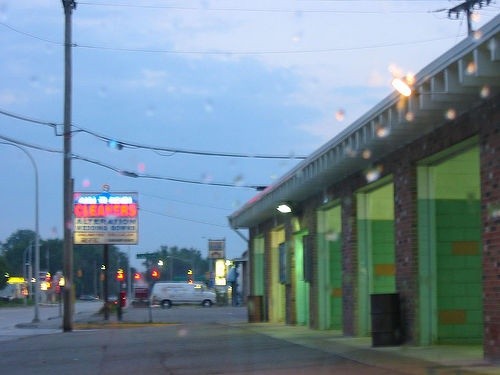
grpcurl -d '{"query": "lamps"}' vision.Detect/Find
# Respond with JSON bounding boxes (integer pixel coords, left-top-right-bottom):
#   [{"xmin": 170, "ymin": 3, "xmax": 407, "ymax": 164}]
[{"xmin": 276, "ymin": 202, "xmax": 296, "ymax": 213}]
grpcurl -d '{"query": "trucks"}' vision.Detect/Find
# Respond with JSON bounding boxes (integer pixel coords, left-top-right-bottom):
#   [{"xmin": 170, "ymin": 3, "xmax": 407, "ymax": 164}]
[{"xmin": 131, "ymin": 284, "xmax": 150, "ymax": 306}]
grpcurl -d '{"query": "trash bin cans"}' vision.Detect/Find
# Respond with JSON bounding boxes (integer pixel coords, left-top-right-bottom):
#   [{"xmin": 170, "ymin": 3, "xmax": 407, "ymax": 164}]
[
  {"xmin": 247, "ymin": 294, "xmax": 263, "ymax": 322},
  {"xmin": 370, "ymin": 292, "xmax": 405, "ymax": 346}
]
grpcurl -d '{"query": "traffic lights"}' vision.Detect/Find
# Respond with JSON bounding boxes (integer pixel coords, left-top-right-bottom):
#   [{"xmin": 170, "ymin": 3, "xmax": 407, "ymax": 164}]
[
  {"xmin": 59, "ymin": 278, "xmax": 65, "ymax": 286},
  {"xmin": 134, "ymin": 274, "xmax": 141, "ymax": 279},
  {"xmin": 116, "ymin": 269, "xmax": 124, "ymax": 279},
  {"xmin": 45, "ymin": 273, "xmax": 51, "ymax": 281},
  {"xmin": 157, "ymin": 259, "xmax": 164, "ymax": 266},
  {"xmin": 151, "ymin": 271, "xmax": 158, "ymax": 278},
  {"xmin": 187, "ymin": 269, "xmax": 193, "ymax": 275},
  {"xmin": 100, "ymin": 265, "xmax": 106, "ymax": 270}
]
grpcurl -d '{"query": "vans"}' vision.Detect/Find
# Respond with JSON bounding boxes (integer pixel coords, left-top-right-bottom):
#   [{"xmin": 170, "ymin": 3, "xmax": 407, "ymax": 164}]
[{"xmin": 150, "ymin": 282, "xmax": 217, "ymax": 309}]
